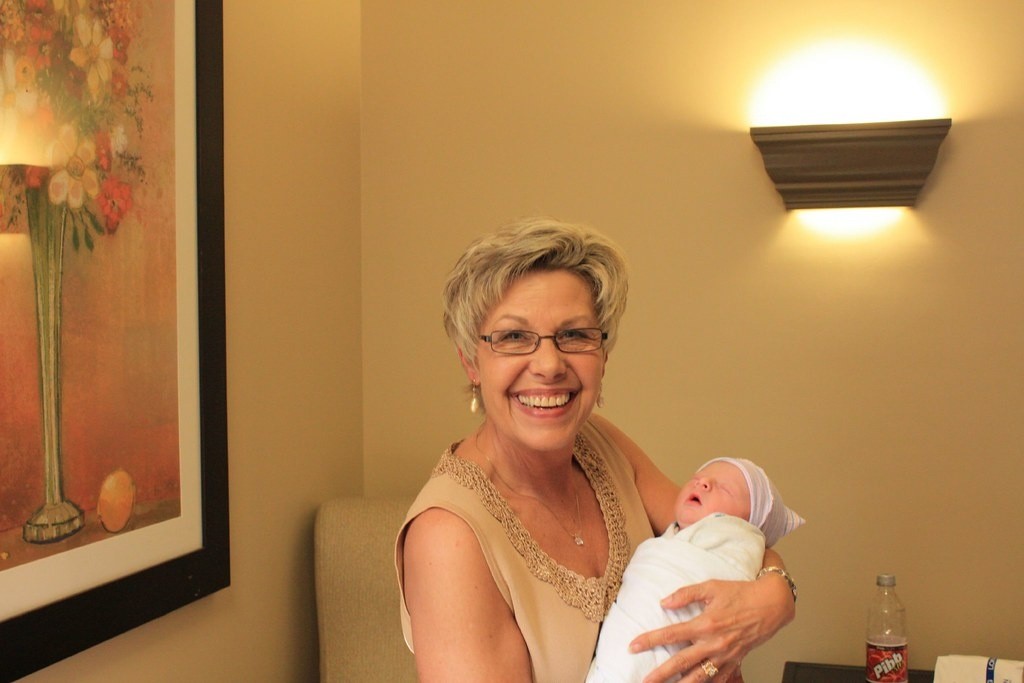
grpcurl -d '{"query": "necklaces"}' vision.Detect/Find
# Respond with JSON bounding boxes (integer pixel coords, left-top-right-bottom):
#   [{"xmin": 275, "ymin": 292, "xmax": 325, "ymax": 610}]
[{"xmin": 475, "ymin": 422, "xmax": 584, "ymax": 547}]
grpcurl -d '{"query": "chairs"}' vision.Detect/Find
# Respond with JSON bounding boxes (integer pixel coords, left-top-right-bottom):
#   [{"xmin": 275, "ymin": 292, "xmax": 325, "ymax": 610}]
[{"xmin": 313, "ymin": 500, "xmax": 421, "ymax": 683}]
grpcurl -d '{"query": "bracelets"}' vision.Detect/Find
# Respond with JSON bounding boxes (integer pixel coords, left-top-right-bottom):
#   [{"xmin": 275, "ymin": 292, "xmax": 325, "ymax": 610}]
[{"xmin": 756, "ymin": 566, "xmax": 798, "ymax": 603}]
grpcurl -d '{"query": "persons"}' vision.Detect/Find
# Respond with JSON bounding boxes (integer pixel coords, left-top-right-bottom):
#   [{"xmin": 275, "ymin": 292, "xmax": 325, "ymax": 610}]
[
  {"xmin": 390, "ymin": 213, "xmax": 794, "ymax": 683},
  {"xmin": 584, "ymin": 457, "xmax": 806, "ymax": 683}
]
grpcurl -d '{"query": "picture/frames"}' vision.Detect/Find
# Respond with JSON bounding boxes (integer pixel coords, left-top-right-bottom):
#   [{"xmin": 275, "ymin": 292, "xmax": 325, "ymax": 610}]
[{"xmin": 0, "ymin": 0, "xmax": 234, "ymax": 683}]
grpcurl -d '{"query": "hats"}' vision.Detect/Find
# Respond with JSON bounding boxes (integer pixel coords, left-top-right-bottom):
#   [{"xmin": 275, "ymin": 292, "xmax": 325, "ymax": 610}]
[{"xmin": 695, "ymin": 457, "xmax": 805, "ymax": 546}]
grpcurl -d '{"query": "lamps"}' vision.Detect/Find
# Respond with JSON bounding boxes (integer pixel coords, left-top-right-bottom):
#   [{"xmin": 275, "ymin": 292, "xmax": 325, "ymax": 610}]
[{"xmin": 750, "ymin": 118, "xmax": 952, "ymax": 211}]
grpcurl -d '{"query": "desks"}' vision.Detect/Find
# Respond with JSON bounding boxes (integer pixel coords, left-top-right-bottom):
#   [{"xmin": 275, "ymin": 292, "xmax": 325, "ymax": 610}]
[{"xmin": 781, "ymin": 660, "xmax": 934, "ymax": 683}]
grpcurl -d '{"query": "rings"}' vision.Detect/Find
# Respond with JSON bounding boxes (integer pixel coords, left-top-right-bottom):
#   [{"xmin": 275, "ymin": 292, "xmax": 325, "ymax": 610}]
[{"xmin": 701, "ymin": 659, "xmax": 719, "ymax": 678}]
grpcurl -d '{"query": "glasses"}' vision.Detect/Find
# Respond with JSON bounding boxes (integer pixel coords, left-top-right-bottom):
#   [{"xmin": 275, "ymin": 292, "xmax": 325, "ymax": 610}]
[{"xmin": 478, "ymin": 328, "xmax": 608, "ymax": 355}]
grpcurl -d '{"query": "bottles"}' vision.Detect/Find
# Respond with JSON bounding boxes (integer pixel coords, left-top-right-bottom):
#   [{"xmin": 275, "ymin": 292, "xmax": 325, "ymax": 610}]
[{"xmin": 864, "ymin": 572, "xmax": 910, "ymax": 683}]
